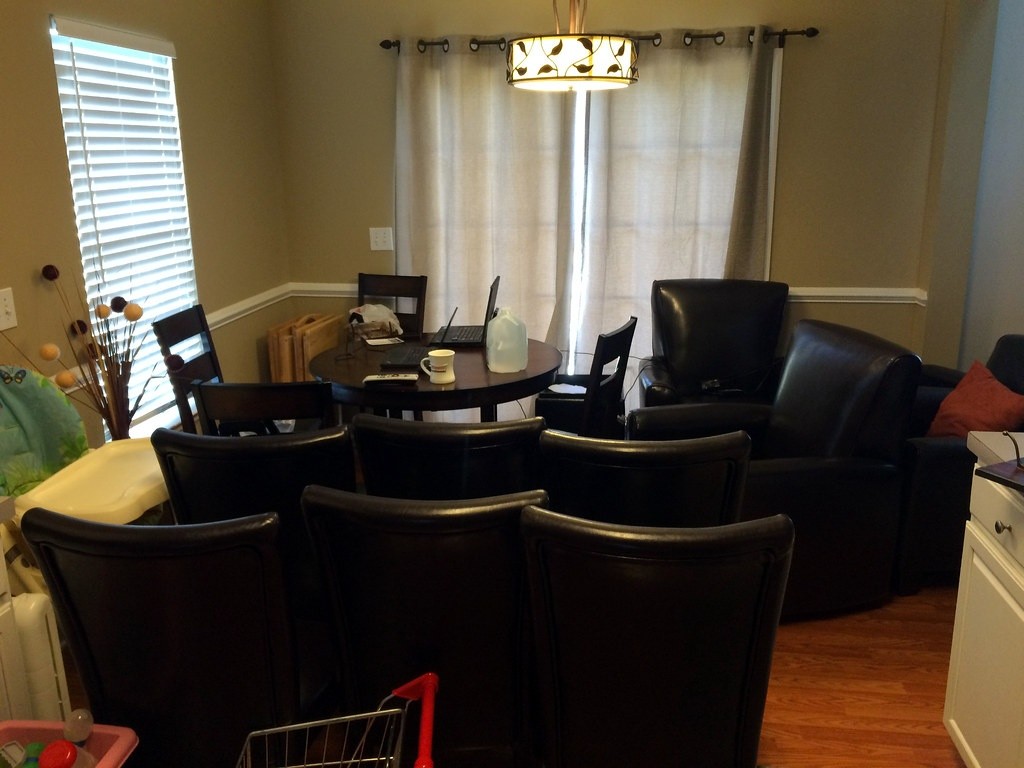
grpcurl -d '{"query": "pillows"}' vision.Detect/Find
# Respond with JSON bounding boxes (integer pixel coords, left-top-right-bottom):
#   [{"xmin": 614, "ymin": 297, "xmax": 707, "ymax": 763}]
[{"xmin": 926, "ymin": 361, "xmax": 1024, "ymax": 438}]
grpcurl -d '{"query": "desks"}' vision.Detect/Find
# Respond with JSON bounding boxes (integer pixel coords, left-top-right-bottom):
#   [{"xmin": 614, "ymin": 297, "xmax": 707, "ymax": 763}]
[{"xmin": 308, "ymin": 332, "xmax": 563, "ymax": 423}]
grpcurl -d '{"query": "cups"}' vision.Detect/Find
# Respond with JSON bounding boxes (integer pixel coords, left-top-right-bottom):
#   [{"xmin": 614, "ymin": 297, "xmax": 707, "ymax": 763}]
[{"xmin": 421, "ymin": 349, "xmax": 456, "ymax": 384}]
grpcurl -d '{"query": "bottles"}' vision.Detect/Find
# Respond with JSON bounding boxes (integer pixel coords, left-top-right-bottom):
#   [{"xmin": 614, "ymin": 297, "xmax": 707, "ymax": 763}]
[{"xmin": 486, "ymin": 307, "xmax": 528, "ymax": 374}]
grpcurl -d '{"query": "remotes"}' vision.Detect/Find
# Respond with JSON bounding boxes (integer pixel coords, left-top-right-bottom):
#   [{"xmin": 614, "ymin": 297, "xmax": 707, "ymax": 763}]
[{"xmin": 361, "ymin": 373, "xmax": 419, "ymax": 384}]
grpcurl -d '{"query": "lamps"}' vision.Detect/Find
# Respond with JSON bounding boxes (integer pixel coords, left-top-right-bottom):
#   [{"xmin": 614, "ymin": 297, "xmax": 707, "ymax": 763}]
[{"xmin": 507, "ymin": 0, "xmax": 640, "ymax": 92}]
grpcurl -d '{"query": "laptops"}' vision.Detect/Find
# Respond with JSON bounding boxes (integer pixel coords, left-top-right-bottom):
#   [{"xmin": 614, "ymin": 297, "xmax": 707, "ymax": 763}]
[
  {"xmin": 385, "ymin": 306, "xmax": 457, "ymax": 369},
  {"xmin": 428, "ymin": 275, "xmax": 499, "ymax": 347}
]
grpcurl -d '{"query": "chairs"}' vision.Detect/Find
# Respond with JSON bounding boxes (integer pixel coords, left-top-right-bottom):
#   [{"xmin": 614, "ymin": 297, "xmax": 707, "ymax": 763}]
[
  {"xmin": 22, "ymin": 509, "xmax": 295, "ymax": 767},
  {"xmin": 191, "ymin": 379, "xmax": 335, "ymax": 436},
  {"xmin": 538, "ymin": 429, "xmax": 752, "ymax": 527},
  {"xmin": 358, "ymin": 272, "xmax": 427, "ymax": 332},
  {"xmin": 299, "ymin": 484, "xmax": 549, "ymax": 767},
  {"xmin": 1, "ymin": 364, "xmax": 86, "ymax": 502},
  {"xmin": 153, "ymin": 305, "xmax": 224, "ymax": 435},
  {"xmin": 535, "ymin": 317, "xmax": 638, "ymax": 439},
  {"xmin": 151, "ymin": 425, "xmax": 356, "ymax": 526},
  {"xmin": 350, "ymin": 412, "xmax": 546, "ymax": 499},
  {"xmin": 521, "ymin": 505, "xmax": 794, "ymax": 768}
]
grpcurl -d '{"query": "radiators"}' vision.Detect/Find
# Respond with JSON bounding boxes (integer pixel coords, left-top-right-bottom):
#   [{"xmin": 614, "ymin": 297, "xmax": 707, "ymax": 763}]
[{"xmin": 12, "ymin": 591, "xmax": 70, "ymax": 720}]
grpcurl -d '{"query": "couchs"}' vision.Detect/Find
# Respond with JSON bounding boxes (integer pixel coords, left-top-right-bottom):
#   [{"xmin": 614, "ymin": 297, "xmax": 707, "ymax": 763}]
[
  {"xmin": 626, "ymin": 320, "xmax": 923, "ymax": 617},
  {"xmin": 638, "ymin": 279, "xmax": 788, "ymax": 408},
  {"xmin": 896, "ymin": 334, "xmax": 1024, "ymax": 597}
]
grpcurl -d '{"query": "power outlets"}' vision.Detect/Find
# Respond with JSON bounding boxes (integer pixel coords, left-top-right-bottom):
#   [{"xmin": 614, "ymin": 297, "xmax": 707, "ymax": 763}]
[
  {"xmin": 0, "ymin": 287, "xmax": 17, "ymax": 332},
  {"xmin": 369, "ymin": 227, "xmax": 393, "ymax": 250}
]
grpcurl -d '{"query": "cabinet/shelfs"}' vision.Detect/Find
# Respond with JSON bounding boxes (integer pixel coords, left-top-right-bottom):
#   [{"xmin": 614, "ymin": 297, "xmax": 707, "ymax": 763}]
[{"xmin": 944, "ymin": 458, "xmax": 1024, "ymax": 768}]
